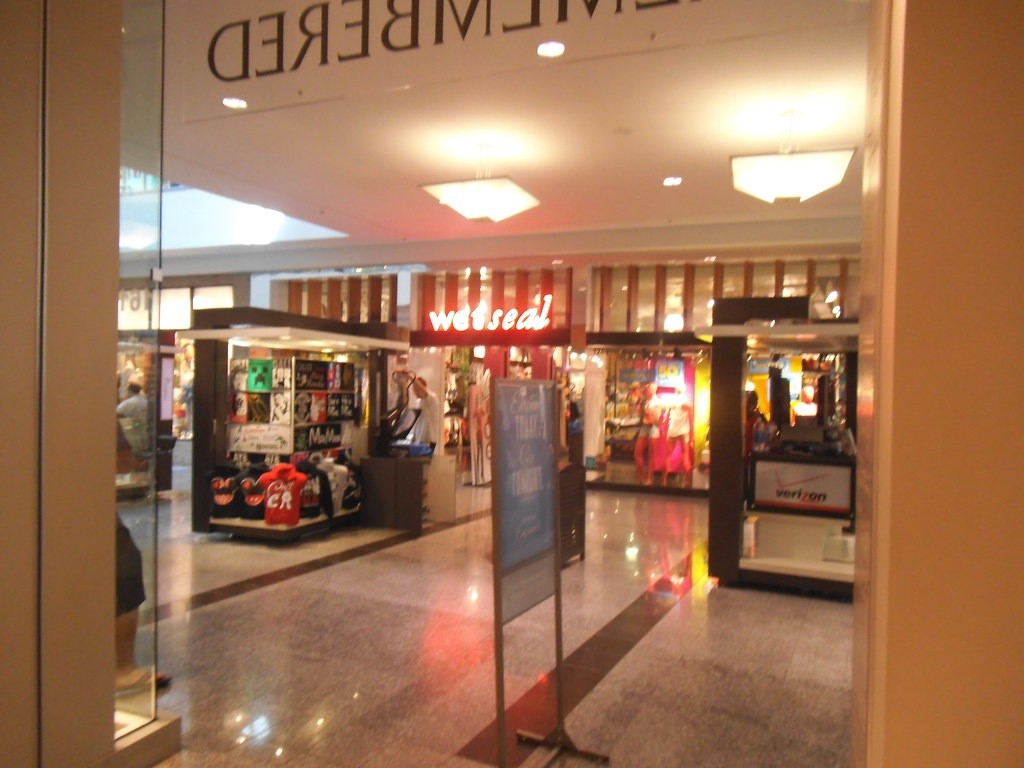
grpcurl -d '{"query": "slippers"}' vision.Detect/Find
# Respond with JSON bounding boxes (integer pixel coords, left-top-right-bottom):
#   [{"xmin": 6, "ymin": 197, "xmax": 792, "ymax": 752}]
[{"xmin": 115, "ymin": 667, "xmax": 172, "ymax": 701}]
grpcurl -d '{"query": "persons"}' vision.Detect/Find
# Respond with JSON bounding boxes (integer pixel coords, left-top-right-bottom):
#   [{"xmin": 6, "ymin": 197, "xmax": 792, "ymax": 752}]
[
  {"xmin": 745, "ymin": 390, "xmax": 766, "ymax": 511},
  {"xmin": 114, "ymin": 420, "xmax": 174, "ymax": 698},
  {"xmin": 115, "ymin": 382, "xmax": 146, "ymax": 423},
  {"xmin": 203, "ymin": 448, "xmax": 364, "ymax": 527},
  {"xmin": 631, "ymin": 381, "xmax": 666, "ymax": 487},
  {"xmin": 396, "ymin": 367, "xmax": 440, "ymax": 455},
  {"xmin": 660, "ymin": 385, "xmax": 694, "ymax": 490}
]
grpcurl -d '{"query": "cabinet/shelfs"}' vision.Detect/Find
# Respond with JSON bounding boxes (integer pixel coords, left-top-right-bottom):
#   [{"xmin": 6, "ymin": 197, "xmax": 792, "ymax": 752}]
[{"xmin": 208, "ymin": 355, "xmax": 368, "ymax": 544}]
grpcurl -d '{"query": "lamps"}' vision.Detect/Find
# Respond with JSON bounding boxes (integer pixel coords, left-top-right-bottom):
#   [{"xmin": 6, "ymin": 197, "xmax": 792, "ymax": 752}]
[
  {"xmin": 420, "ymin": 174, "xmax": 542, "ymax": 224},
  {"xmin": 730, "ymin": 149, "xmax": 856, "ymax": 203}
]
